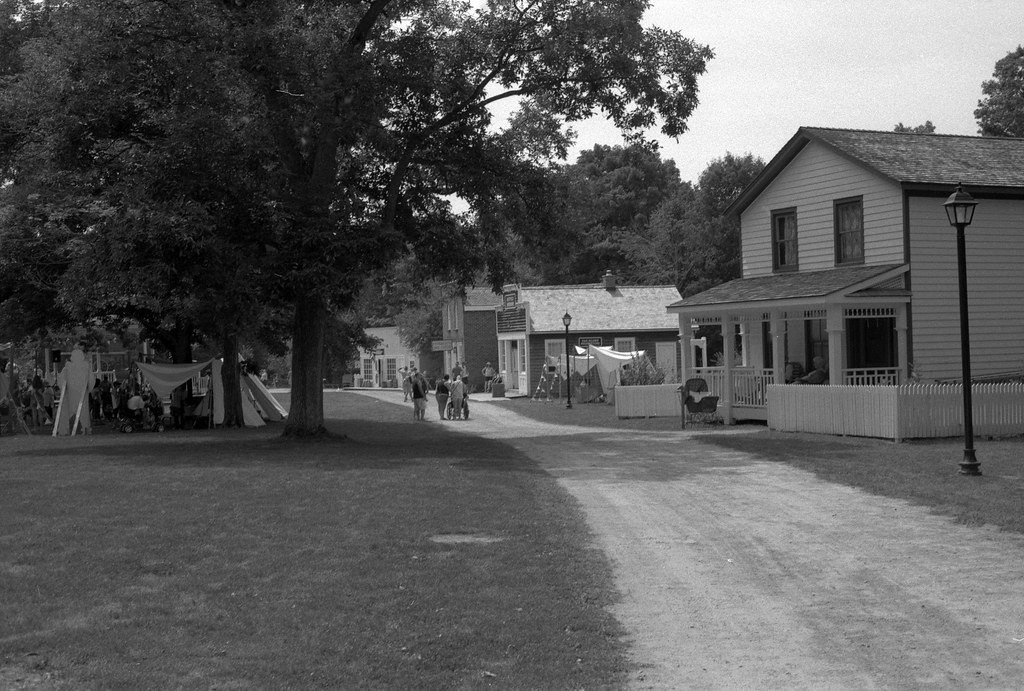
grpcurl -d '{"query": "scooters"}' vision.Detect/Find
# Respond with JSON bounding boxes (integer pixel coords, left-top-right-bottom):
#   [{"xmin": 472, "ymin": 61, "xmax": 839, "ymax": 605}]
[{"xmin": 119, "ymin": 409, "xmax": 166, "ymax": 434}]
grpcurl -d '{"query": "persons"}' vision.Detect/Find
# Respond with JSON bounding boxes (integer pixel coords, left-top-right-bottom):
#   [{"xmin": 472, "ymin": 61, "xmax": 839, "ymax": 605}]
[
  {"xmin": 260, "ymin": 369, "xmax": 268, "ymax": 389},
  {"xmin": 482, "ymin": 362, "xmax": 494, "ymax": 393},
  {"xmin": 799, "ymin": 356, "xmax": 825, "ymax": 384},
  {"xmin": 25, "ymin": 375, "xmax": 186, "ymax": 434},
  {"xmin": 397, "ymin": 361, "xmax": 469, "ymax": 421}
]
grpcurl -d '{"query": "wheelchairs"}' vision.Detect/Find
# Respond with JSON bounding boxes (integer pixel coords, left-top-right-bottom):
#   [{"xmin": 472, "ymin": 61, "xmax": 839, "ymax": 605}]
[{"xmin": 446, "ymin": 394, "xmax": 470, "ymax": 421}]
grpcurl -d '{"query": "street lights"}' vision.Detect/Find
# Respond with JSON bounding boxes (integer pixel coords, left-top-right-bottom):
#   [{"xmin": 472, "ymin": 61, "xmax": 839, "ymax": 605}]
[
  {"xmin": 562, "ymin": 310, "xmax": 574, "ymax": 410},
  {"xmin": 943, "ymin": 180, "xmax": 983, "ymax": 477}
]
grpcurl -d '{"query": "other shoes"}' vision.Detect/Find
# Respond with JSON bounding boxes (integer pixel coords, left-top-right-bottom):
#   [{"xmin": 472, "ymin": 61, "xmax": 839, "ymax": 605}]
[
  {"xmin": 112, "ymin": 423, "xmax": 116, "ymax": 428},
  {"xmin": 452, "ymin": 418, "xmax": 456, "ymax": 420},
  {"xmin": 100, "ymin": 423, "xmax": 106, "ymax": 425},
  {"xmin": 458, "ymin": 418, "xmax": 462, "ymax": 420},
  {"xmin": 440, "ymin": 417, "xmax": 446, "ymax": 420}
]
